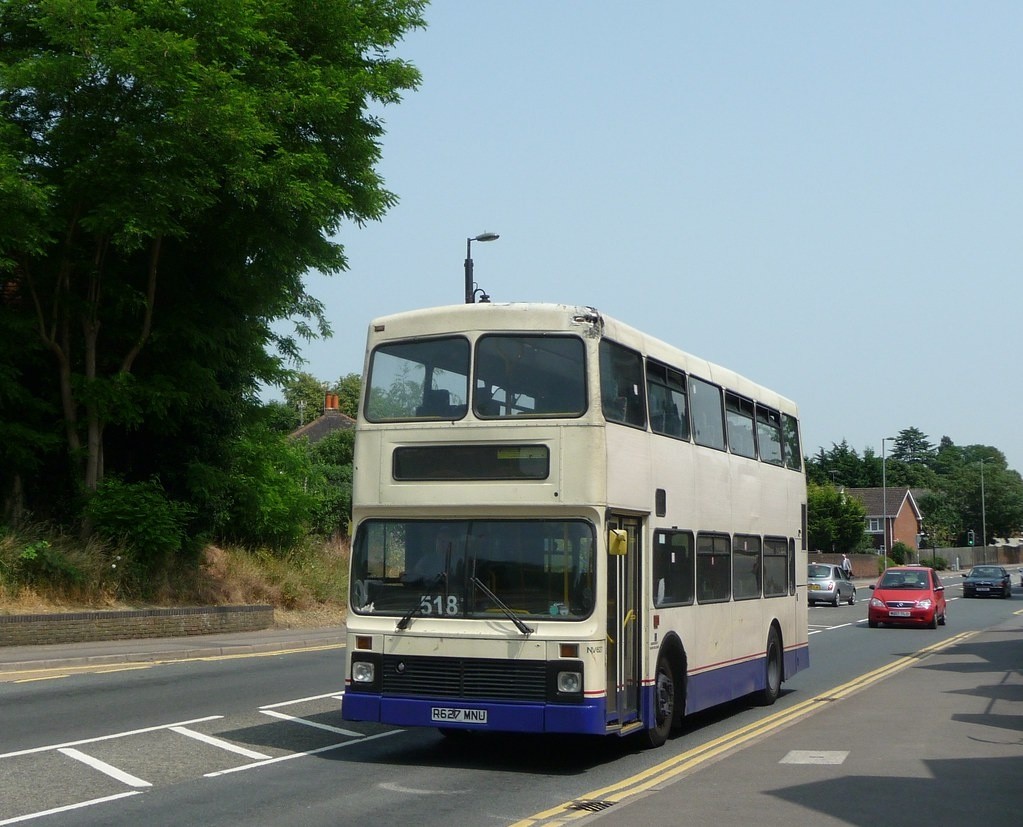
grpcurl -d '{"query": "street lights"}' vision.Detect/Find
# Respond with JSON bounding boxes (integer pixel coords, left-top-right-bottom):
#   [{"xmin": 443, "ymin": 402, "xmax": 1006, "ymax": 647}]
[
  {"xmin": 883, "ymin": 436, "xmax": 896, "ymax": 573},
  {"xmin": 981, "ymin": 455, "xmax": 995, "ymax": 567},
  {"xmin": 465, "ymin": 231, "xmax": 499, "ymax": 304}
]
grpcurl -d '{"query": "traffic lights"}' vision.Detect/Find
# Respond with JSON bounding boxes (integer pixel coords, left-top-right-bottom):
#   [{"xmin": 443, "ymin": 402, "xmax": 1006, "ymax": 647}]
[{"xmin": 968, "ymin": 532, "xmax": 974, "ymax": 546}]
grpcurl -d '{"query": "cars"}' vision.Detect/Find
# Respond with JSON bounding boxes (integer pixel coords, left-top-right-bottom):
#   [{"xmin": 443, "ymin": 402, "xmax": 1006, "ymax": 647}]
[
  {"xmin": 867, "ymin": 564, "xmax": 946, "ymax": 628},
  {"xmin": 961, "ymin": 565, "xmax": 1012, "ymax": 599}
]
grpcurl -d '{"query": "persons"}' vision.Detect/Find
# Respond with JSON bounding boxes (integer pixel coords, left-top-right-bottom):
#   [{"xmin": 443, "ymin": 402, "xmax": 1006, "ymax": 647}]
[
  {"xmin": 411, "ymin": 530, "xmax": 488, "ymax": 597},
  {"xmin": 753, "ymin": 555, "xmax": 767, "ymax": 594},
  {"xmin": 841, "ymin": 554, "xmax": 852, "ymax": 577}
]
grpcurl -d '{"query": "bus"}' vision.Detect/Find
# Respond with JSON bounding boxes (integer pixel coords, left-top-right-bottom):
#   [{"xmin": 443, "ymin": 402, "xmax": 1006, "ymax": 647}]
[
  {"xmin": 338, "ymin": 299, "xmax": 812, "ymax": 750},
  {"xmin": 807, "ymin": 563, "xmax": 856, "ymax": 607}
]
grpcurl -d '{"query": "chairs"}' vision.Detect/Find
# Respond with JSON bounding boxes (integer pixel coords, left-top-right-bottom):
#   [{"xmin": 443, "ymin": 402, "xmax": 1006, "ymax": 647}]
[
  {"xmin": 416, "ymin": 389, "xmax": 458, "ymax": 417},
  {"xmin": 458, "ymin": 387, "xmax": 500, "ymax": 417}
]
